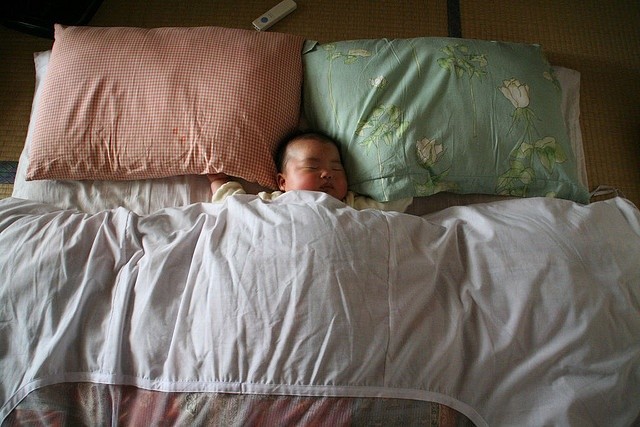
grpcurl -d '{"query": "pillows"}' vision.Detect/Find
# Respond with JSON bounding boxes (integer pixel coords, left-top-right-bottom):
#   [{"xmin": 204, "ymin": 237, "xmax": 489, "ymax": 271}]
[
  {"xmin": 304, "ymin": 34, "xmax": 593, "ymax": 204},
  {"xmin": 24, "ymin": 23, "xmax": 304, "ymax": 191}
]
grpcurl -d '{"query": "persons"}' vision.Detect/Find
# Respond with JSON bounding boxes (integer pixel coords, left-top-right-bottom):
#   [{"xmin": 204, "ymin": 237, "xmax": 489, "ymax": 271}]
[{"xmin": 206, "ymin": 129, "xmax": 414, "ymax": 211}]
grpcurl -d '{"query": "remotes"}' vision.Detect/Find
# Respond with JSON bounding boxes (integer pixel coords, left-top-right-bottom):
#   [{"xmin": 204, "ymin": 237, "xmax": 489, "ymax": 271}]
[{"xmin": 251, "ymin": 0, "xmax": 297, "ymax": 31}]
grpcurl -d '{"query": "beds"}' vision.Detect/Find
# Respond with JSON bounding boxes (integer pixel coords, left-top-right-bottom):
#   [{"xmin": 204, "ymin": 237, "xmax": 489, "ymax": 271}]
[{"xmin": 1, "ymin": 1, "xmax": 638, "ymax": 427}]
